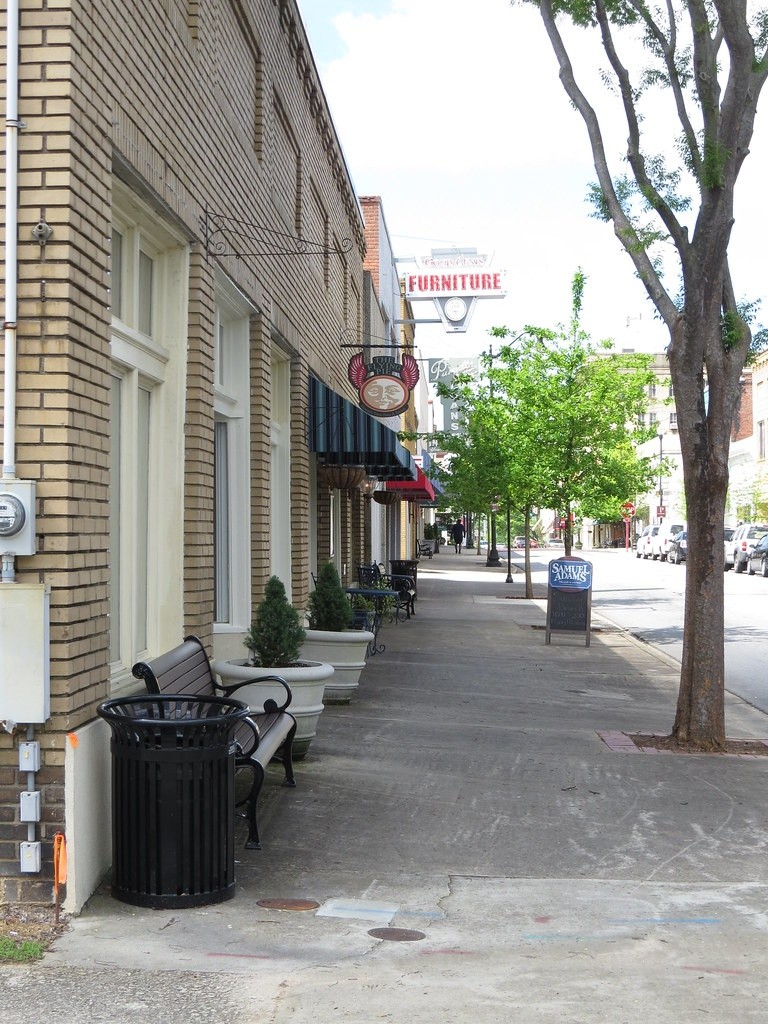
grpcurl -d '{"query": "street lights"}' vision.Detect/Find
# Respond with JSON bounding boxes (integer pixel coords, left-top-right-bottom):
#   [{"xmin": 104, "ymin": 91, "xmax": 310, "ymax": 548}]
[
  {"xmin": 655, "ymin": 422, "xmax": 669, "ymax": 523},
  {"xmin": 483, "ymin": 328, "xmax": 547, "ymax": 569}
]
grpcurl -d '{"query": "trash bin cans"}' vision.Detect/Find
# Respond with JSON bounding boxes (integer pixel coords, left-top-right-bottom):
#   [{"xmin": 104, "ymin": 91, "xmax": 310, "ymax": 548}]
[
  {"xmin": 96, "ymin": 694, "xmax": 251, "ymax": 909},
  {"xmin": 390, "ymin": 560, "xmax": 417, "ymax": 602},
  {"xmin": 433, "ymin": 538, "xmax": 440, "ymax": 554}
]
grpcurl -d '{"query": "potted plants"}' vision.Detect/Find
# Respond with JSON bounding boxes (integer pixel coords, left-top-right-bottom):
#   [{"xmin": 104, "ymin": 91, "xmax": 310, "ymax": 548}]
[
  {"xmin": 301, "ymin": 563, "xmax": 375, "ymax": 704},
  {"xmin": 211, "ymin": 574, "xmax": 334, "ymax": 762}
]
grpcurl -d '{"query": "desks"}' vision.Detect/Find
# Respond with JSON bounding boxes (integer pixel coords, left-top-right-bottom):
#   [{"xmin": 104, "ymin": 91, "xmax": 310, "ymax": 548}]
[
  {"xmin": 343, "ymin": 586, "xmax": 399, "ymax": 633},
  {"xmin": 382, "ymin": 574, "xmax": 414, "ymax": 620}
]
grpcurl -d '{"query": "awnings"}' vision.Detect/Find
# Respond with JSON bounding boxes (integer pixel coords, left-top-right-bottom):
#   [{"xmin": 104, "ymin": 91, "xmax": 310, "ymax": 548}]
[{"xmin": 310, "ymin": 377, "xmax": 438, "ymax": 502}]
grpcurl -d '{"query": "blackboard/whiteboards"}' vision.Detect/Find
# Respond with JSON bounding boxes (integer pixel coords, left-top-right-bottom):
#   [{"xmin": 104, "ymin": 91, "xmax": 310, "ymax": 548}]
[{"xmin": 546, "ymin": 584, "xmax": 591, "ymax": 635}]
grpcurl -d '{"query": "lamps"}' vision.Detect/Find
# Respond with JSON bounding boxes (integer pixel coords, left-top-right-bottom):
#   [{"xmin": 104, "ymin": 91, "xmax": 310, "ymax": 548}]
[{"xmin": 360, "ymin": 473, "xmax": 379, "ymax": 507}]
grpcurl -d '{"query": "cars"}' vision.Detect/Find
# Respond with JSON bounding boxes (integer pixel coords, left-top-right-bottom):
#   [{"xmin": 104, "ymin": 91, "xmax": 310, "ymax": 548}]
[
  {"xmin": 545, "ymin": 539, "xmax": 564, "ymax": 548},
  {"xmin": 668, "ymin": 530, "xmax": 688, "ymax": 564},
  {"xmin": 746, "ymin": 533, "xmax": 768, "ymax": 578},
  {"xmin": 512, "ymin": 536, "xmax": 537, "ymax": 548}
]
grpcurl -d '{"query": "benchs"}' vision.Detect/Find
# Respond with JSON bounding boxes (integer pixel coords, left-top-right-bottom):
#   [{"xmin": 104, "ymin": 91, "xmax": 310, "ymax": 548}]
[
  {"xmin": 415, "ymin": 539, "xmax": 433, "ymax": 561},
  {"xmin": 130, "ymin": 636, "xmax": 297, "ymax": 850}
]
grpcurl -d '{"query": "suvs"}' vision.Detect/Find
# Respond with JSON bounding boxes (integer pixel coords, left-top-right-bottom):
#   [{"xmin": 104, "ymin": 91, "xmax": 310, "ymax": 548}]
[
  {"xmin": 636, "ymin": 520, "xmax": 686, "ymax": 562},
  {"xmin": 724, "ymin": 522, "xmax": 768, "ymax": 573}
]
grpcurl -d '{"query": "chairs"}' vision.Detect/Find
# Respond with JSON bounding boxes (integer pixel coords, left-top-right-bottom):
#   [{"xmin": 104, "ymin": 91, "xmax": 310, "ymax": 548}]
[
  {"xmin": 357, "ymin": 560, "xmax": 415, "ymax": 624},
  {"xmin": 309, "ymin": 572, "xmax": 387, "ymax": 658}
]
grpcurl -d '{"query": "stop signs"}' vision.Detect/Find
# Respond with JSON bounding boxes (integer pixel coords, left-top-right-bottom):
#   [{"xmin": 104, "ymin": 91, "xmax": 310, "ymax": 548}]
[
  {"xmin": 559, "ymin": 518, "xmax": 566, "ymax": 530},
  {"xmin": 618, "ymin": 502, "xmax": 636, "ymax": 518}
]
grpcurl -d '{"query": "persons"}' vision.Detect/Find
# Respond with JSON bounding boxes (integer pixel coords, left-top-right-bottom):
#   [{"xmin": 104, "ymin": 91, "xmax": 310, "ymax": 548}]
[{"xmin": 450, "ymin": 519, "xmax": 465, "ymax": 554}]
